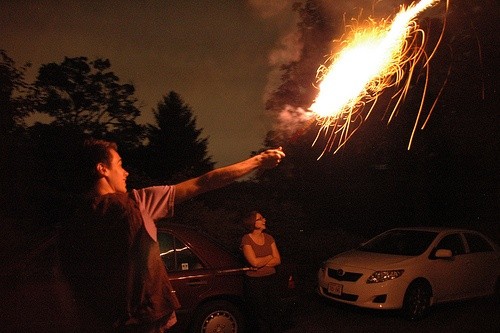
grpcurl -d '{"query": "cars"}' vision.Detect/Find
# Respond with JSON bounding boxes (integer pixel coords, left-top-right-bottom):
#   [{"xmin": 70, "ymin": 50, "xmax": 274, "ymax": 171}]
[
  {"xmin": 0, "ymin": 218, "xmax": 299, "ymax": 333},
  {"xmin": 316, "ymin": 225, "xmax": 500, "ymax": 322}
]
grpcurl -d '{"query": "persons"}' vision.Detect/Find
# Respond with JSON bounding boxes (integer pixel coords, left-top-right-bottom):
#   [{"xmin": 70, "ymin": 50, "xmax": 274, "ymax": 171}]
[
  {"xmin": 57, "ymin": 140, "xmax": 285, "ymax": 333},
  {"xmin": 238, "ymin": 210, "xmax": 282, "ymax": 333}
]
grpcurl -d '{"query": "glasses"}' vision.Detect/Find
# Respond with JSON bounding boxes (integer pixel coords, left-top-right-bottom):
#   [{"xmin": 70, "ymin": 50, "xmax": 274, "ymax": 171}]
[{"xmin": 255, "ymin": 217, "xmax": 264, "ymax": 221}]
